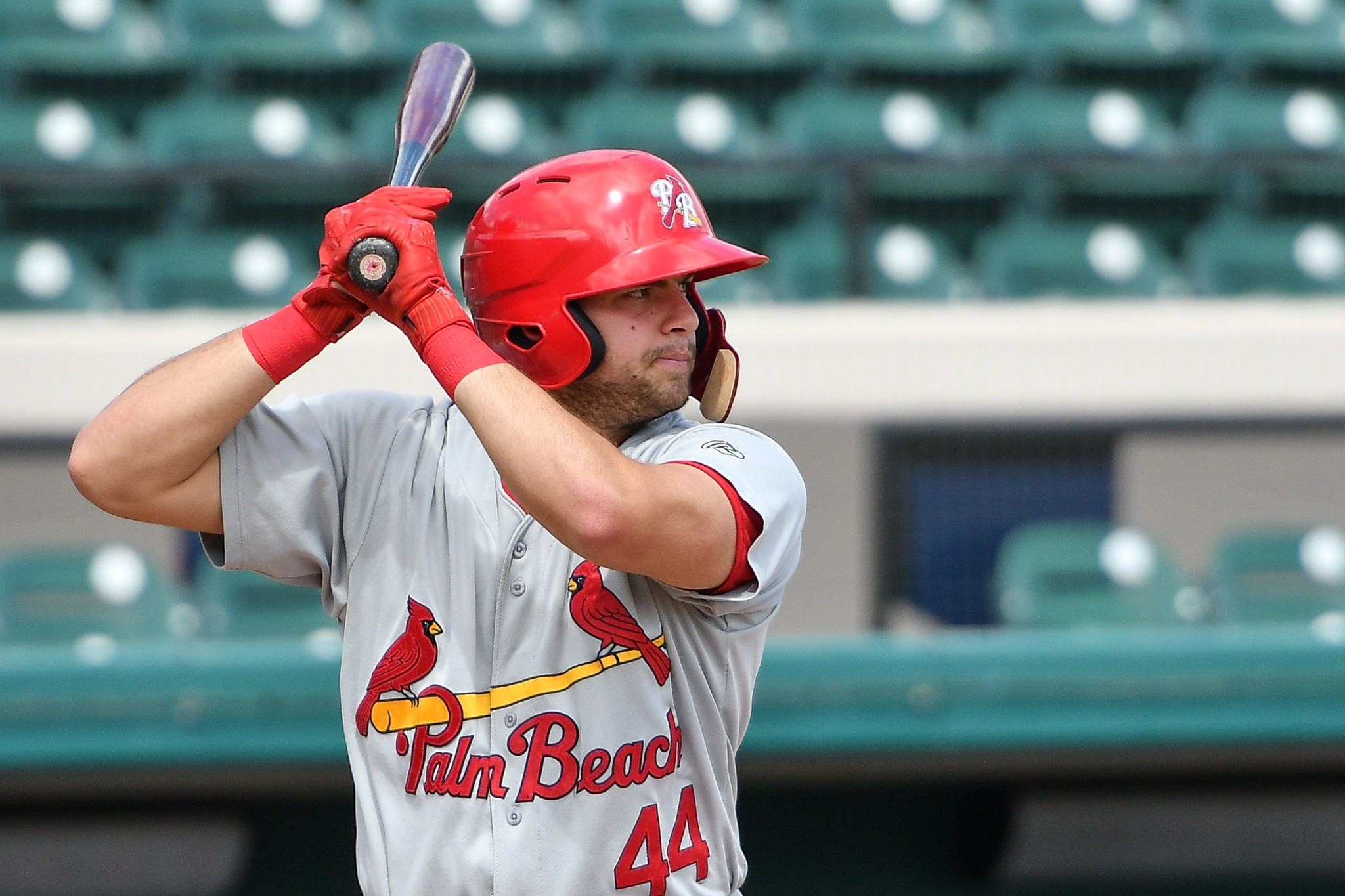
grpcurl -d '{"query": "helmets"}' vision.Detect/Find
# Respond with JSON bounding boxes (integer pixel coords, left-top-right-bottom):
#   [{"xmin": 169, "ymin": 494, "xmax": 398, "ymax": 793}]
[{"xmin": 460, "ymin": 149, "xmax": 768, "ymax": 423}]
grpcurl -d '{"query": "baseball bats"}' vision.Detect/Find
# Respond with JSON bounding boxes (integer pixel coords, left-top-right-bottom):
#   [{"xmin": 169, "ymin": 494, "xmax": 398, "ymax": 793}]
[{"xmin": 347, "ymin": 38, "xmax": 479, "ymax": 295}]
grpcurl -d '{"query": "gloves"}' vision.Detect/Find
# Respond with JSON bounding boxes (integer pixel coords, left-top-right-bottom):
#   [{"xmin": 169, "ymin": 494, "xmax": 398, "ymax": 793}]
[
  {"xmin": 303, "ymin": 207, "xmax": 474, "ymax": 359},
  {"xmin": 291, "ymin": 185, "xmax": 452, "ymax": 344}
]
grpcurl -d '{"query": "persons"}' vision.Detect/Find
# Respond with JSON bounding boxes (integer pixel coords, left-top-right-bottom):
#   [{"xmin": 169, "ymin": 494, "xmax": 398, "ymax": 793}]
[{"xmin": 67, "ymin": 150, "xmax": 807, "ymax": 896}]
